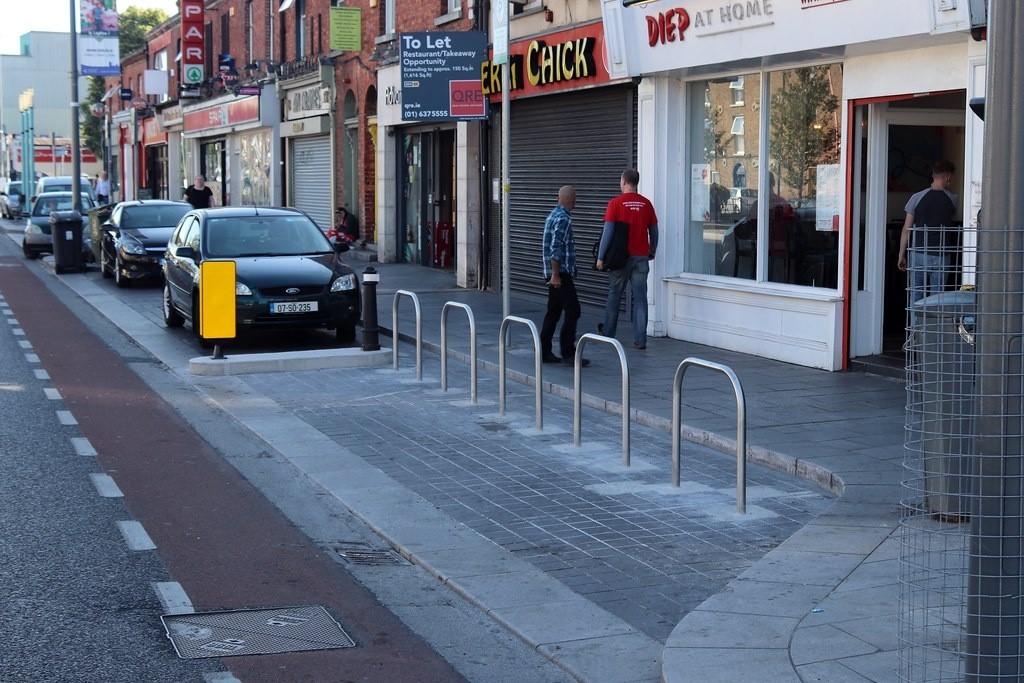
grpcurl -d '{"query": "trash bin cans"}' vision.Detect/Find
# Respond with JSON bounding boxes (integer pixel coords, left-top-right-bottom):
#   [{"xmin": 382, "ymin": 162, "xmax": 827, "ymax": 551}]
[
  {"xmin": 48, "ymin": 209, "xmax": 83, "ymax": 274},
  {"xmin": 913, "ymin": 292, "xmax": 978, "ymax": 523}
]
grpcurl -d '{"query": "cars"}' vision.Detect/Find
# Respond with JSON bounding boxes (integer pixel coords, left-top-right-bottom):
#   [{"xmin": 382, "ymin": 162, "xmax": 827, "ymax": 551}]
[
  {"xmin": 161, "ymin": 206, "xmax": 363, "ymax": 349},
  {"xmin": 720, "ymin": 196, "xmax": 839, "ymax": 279},
  {"xmin": 20, "ymin": 191, "xmax": 96, "ymax": 260},
  {"xmin": 99, "ymin": 198, "xmax": 196, "ymax": 288},
  {"xmin": 0, "ymin": 180, "xmax": 37, "ymax": 222}
]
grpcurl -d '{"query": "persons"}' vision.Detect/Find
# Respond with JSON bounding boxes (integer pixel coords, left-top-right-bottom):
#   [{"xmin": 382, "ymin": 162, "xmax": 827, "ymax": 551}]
[
  {"xmin": 897, "ymin": 159, "xmax": 961, "ymax": 339},
  {"xmin": 184, "ymin": 175, "xmax": 217, "ymax": 209},
  {"xmin": 93, "ymin": 3, "xmax": 105, "ymax": 33},
  {"xmin": 45, "ymin": 199, "xmax": 60, "ymax": 215},
  {"xmin": 328, "ymin": 208, "xmax": 360, "ymax": 253},
  {"xmin": 598, "ymin": 170, "xmax": 659, "ymax": 351},
  {"xmin": 93, "ymin": 170, "xmax": 120, "ymax": 207},
  {"xmin": 541, "ymin": 186, "xmax": 590, "ymax": 366},
  {"xmin": 734, "ymin": 170, "xmax": 791, "ymax": 283}
]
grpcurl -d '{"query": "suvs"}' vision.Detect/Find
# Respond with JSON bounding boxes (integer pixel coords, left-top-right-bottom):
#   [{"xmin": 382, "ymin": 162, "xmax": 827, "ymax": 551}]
[{"xmin": 30, "ymin": 176, "xmax": 104, "ymax": 215}]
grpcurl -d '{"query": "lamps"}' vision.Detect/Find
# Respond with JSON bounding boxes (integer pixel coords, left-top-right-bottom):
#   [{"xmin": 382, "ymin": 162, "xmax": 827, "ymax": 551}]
[
  {"xmin": 118, "ymin": 88, "xmax": 133, "ymax": 101},
  {"xmin": 968, "ymin": 0, "xmax": 989, "ymax": 42},
  {"xmin": 181, "ymin": 82, "xmax": 212, "ymax": 99}
]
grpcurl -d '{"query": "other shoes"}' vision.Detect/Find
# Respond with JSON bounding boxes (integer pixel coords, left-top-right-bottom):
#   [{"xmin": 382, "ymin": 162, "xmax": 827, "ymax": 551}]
[
  {"xmin": 632, "ymin": 342, "xmax": 647, "ymax": 349},
  {"xmin": 597, "ymin": 322, "xmax": 615, "ymax": 341},
  {"xmin": 542, "ymin": 353, "xmax": 563, "ymax": 364},
  {"xmin": 562, "ymin": 358, "xmax": 592, "ymax": 367}
]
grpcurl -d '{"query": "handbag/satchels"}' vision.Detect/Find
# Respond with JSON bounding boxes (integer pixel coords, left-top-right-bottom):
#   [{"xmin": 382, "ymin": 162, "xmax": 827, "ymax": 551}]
[{"xmin": 592, "ymin": 220, "xmax": 629, "ymax": 270}]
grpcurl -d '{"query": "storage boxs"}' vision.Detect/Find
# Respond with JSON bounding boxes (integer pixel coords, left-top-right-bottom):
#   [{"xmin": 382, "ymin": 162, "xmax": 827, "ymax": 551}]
[{"xmin": 425, "ymin": 218, "xmax": 452, "ymax": 269}]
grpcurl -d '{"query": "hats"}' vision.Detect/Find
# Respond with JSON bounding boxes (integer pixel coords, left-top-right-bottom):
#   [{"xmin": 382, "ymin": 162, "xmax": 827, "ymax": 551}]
[{"xmin": 335, "ymin": 208, "xmax": 345, "ymax": 213}]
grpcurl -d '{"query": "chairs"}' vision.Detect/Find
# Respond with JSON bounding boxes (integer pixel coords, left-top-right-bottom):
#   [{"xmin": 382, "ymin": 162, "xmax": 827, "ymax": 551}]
[
  {"xmin": 733, "ymin": 204, "xmax": 839, "ymax": 288},
  {"xmin": 209, "ymin": 220, "xmax": 241, "ymax": 246},
  {"xmin": 263, "ymin": 218, "xmax": 303, "ymax": 252}
]
